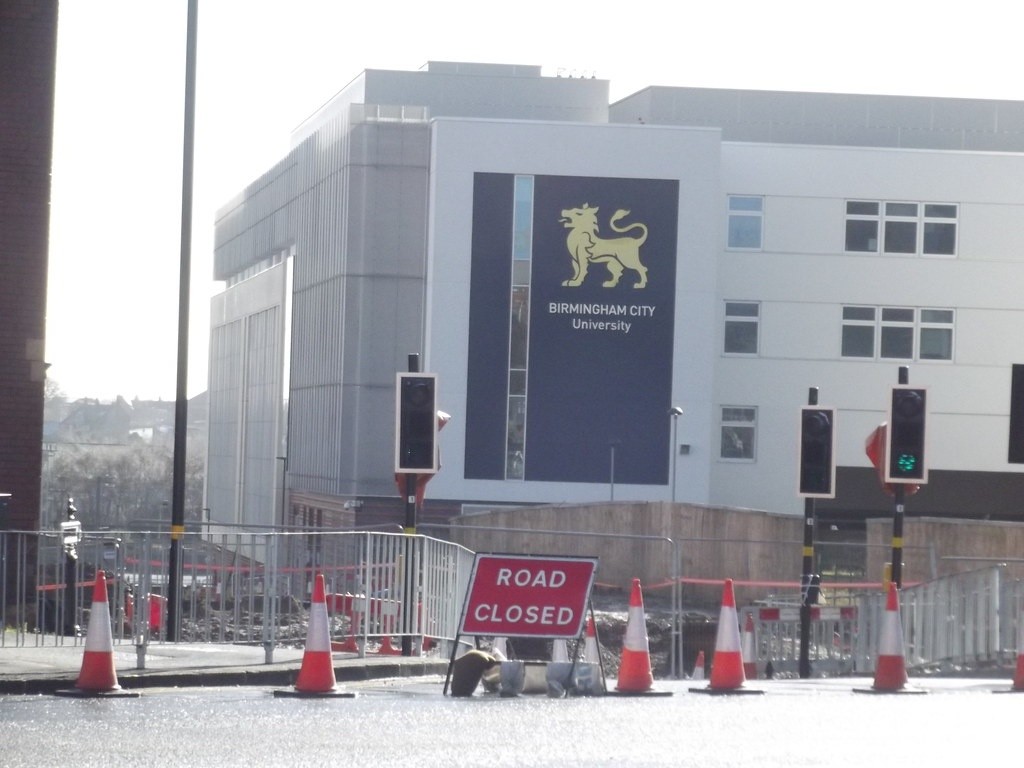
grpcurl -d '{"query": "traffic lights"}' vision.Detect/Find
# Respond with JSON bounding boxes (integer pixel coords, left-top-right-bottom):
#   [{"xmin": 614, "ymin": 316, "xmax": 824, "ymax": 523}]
[
  {"xmin": 795, "ymin": 404, "xmax": 837, "ymax": 500},
  {"xmin": 393, "ymin": 372, "xmax": 439, "ymax": 476},
  {"xmin": 885, "ymin": 383, "xmax": 929, "ymax": 484}
]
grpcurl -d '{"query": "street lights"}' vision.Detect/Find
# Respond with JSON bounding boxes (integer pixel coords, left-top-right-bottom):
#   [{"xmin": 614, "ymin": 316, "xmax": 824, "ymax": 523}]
[
  {"xmin": 667, "ymin": 406, "xmax": 683, "ymax": 503},
  {"xmin": 157, "ymin": 500, "xmax": 168, "ymax": 539},
  {"xmin": 606, "ymin": 438, "xmax": 621, "ymax": 502}
]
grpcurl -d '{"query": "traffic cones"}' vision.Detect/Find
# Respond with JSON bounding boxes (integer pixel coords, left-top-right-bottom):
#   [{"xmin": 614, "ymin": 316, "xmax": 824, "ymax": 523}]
[
  {"xmin": 584, "ymin": 617, "xmax": 600, "ymax": 664},
  {"xmin": 990, "ymin": 611, "xmax": 1024, "ymax": 693},
  {"xmin": 742, "ymin": 611, "xmax": 758, "ymax": 680},
  {"xmin": 53, "ymin": 568, "xmax": 142, "ymax": 698},
  {"xmin": 851, "ymin": 583, "xmax": 928, "ymax": 694},
  {"xmin": 688, "ymin": 578, "xmax": 767, "ymax": 694},
  {"xmin": 600, "ymin": 579, "xmax": 677, "ymax": 696},
  {"xmin": 691, "ymin": 649, "xmax": 706, "ymax": 681},
  {"xmin": 271, "ymin": 573, "xmax": 359, "ymax": 699}
]
